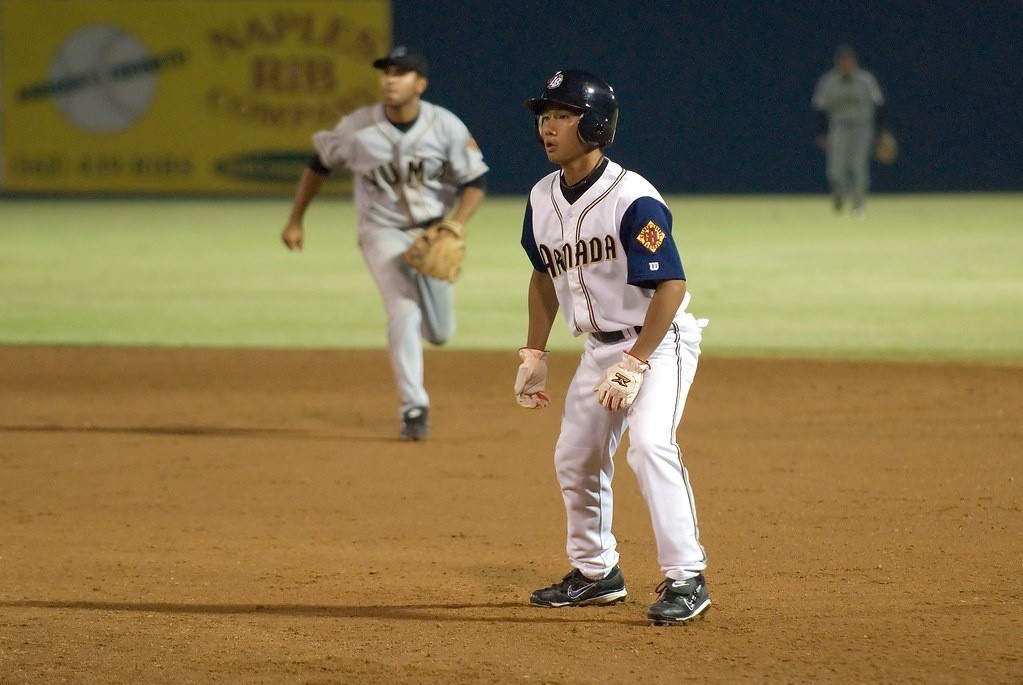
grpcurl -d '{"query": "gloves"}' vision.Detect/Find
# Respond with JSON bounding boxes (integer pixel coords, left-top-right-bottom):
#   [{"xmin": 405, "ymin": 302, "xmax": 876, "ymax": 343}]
[
  {"xmin": 593, "ymin": 349, "xmax": 651, "ymax": 414},
  {"xmin": 514, "ymin": 347, "xmax": 550, "ymax": 409}
]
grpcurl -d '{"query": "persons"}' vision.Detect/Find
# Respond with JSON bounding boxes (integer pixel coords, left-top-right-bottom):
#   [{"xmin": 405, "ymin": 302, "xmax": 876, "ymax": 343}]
[
  {"xmin": 812, "ymin": 44, "xmax": 897, "ymax": 218},
  {"xmin": 283, "ymin": 45, "xmax": 491, "ymax": 440},
  {"xmin": 513, "ymin": 70, "xmax": 711, "ymax": 624}
]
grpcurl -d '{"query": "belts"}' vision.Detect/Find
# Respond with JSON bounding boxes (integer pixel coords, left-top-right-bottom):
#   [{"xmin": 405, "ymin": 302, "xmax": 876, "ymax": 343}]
[
  {"xmin": 592, "ymin": 325, "xmax": 642, "ymax": 343},
  {"xmin": 400, "ymin": 217, "xmax": 443, "ymax": 231}
]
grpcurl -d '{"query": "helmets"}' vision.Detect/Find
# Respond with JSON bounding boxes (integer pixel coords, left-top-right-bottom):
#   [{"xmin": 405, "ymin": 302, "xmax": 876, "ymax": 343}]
[{"xmin": 526, "ymin": 68, "xmax": 618, "ymax": 149}]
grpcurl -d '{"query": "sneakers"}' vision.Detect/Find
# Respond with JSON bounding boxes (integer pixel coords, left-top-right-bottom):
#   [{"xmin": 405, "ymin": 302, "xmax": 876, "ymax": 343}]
[
  {"xmin": 400, "ymin": 407, "xmax": 429, "ymax": 441},
  {"xmin": 530, "ymin": 564, "xmax": 627, "ymax": 608},
  {"xmin": 647, "ymin": 573, "xmax": 711, "ymax": 626}
]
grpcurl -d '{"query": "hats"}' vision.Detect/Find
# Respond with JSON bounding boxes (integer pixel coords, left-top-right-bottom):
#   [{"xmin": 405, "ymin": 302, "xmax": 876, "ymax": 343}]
[{"xmin": 374, "ymin": 46, "xmax": 428, "ymax": 78}]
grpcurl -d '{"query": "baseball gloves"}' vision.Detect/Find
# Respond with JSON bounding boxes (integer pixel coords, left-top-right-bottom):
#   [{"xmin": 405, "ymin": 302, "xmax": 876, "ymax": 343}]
[
  {"xmin": 403, "ymin": 218, "xmax": 466, "ymax": 285},
  {"xmin": 872, "ymin": 131, "xmax": 897, "ymax": 161}
]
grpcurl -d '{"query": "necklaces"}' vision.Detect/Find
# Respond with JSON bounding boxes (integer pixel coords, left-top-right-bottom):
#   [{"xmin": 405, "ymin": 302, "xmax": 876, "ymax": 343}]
[{"xmin": 560, "ymin": 156, "xmax": 603, "ymax": 189}]
